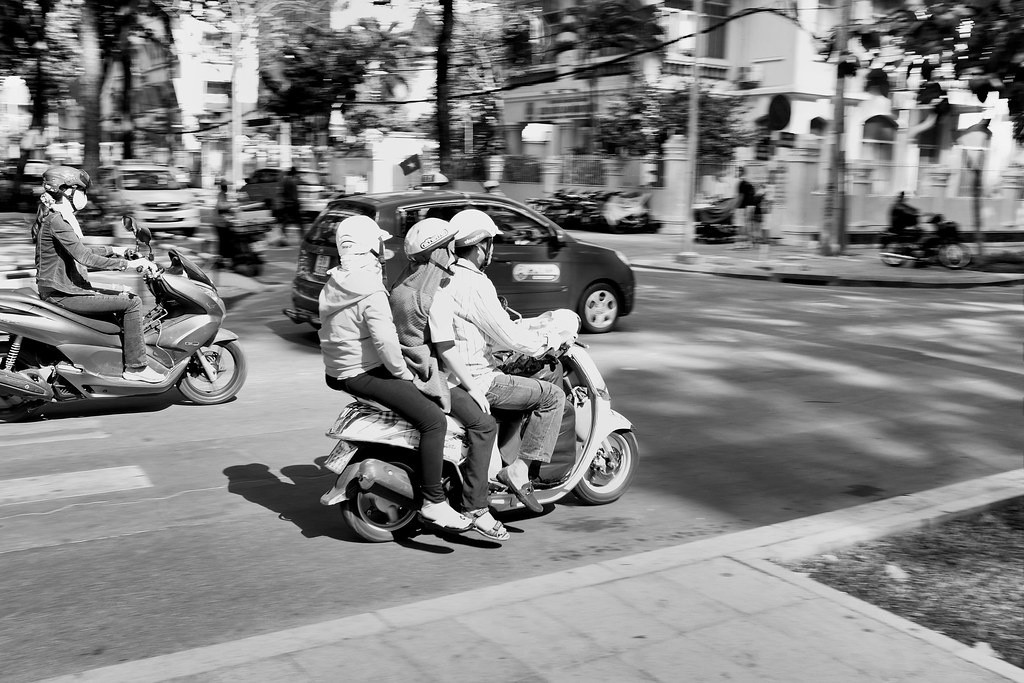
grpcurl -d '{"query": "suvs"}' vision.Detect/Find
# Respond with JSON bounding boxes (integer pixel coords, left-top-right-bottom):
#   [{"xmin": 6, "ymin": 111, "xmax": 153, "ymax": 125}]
[{"xmin": 281, "ymin": 189, "xmax": 636, "ymax": 344}]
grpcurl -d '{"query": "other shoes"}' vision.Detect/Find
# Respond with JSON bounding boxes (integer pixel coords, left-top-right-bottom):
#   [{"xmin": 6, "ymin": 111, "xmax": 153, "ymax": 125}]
[
  {"xmin": 123, "ymin": 366, "xmax": 166, "ymax": 383},
  {"xmin": 417, "ymin": 499, "xmax": 474, "ymax": 532}
]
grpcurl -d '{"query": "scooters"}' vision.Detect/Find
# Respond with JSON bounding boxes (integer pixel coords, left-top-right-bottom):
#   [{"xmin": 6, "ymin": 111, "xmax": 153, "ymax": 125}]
[
  {"xmin": 877, "ymin": 192, "xmax": 973, "ymax": 270},
  {"xmin": 690, "ymin": 177, "xmax": 767, "ymax": 246},
  {"xmin": 213, "ymin": 199, "xmax": 280, "ymax": 278},
  {"xmin": 319, "ymin": 294, "xmax": 640, "ymax": 545},
  {"xmin": 524, "ymin": 188, "xmax": 662, "ymax": 235}
]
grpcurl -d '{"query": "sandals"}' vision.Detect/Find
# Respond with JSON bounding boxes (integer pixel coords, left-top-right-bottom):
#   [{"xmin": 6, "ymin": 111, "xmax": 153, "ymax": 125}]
[{"xmin": 461, "ymin": 507, "xmax": 510, "ymax": 541}]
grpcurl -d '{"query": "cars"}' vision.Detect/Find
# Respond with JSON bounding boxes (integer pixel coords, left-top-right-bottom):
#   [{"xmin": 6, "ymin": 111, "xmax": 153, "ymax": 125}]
[
  {"xmin": 240, "ymin": 168, "xmax": 338, "ymax": 224},
  {"xmin": 0, "ymin": 156, "xmax": 57, "ymax": 212},
  {"xmin": 100, "ymin": 157, "xmax": 205, "ymax": 236}
]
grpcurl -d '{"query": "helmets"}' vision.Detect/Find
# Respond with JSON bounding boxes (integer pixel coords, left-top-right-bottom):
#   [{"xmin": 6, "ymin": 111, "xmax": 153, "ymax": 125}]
[
  {"xmin": 336, "ymin": 214, "xmax": 393, "ymax": 257},
  {"xmin": 42, "ymin": 166, "xmax": 91, "ymax": 193},
  {"xmin": 449, "ymin": 209, "xmax": 504, "ymax": 248},
  {"xmin": 404, "ymin": 218, "xmax": 459, "ymax": 261}
]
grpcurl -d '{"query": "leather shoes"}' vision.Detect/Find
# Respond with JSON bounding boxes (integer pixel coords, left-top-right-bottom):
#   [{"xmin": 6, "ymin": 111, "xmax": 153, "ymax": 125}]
[{"xmin": 496, "ymin": 466, "xmax": 543, "ymax": 512}]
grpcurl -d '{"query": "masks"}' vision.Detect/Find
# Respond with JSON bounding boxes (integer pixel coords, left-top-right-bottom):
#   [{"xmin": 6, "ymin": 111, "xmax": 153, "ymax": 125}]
[{"xmin": 65, "ymin": 189, "xmax": 87, "ymax": 210}]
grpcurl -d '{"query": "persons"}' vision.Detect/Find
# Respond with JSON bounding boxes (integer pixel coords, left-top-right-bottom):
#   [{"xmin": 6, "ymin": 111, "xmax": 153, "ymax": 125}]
[
  {"xmin": 390, "ymin": 218, "xmax": 510, "ymax": 541},
  {"xmin": 317, "ymin": 215, "xmax": 474, "ymax": 533},
  {"xmin": 890, "ymin": 190, "xmax": 935, "ymax": 269},
  {"xmin": 734, "ymin": 165, "xmax": 754, "ymax": 207},
  {"xmin": 447, "ymin": 209, "xmax": 567, "ymax": 513},
  {"xmin": 35, "ymin": 166, "xmax": 166, "ymax": 383},
  {"xmin": 278, "ymin": 166, "xmax": 306, "ymax": 246},
  {"xmin": 214, "ymin": 182, "xmax": 238, "ymax": 268}
]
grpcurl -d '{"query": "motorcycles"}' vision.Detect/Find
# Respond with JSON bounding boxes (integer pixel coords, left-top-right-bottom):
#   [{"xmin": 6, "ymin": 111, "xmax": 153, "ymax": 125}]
[{"xmin": 0, "ymin": 215, "xmax": 246, "ymax": 422}]
